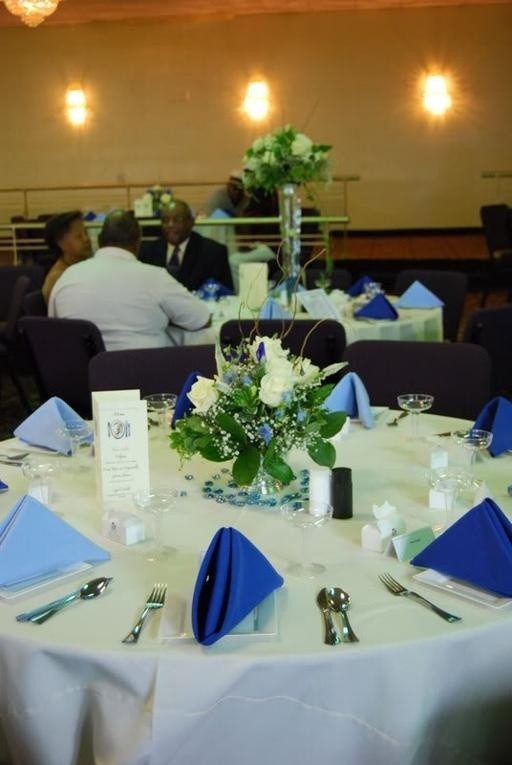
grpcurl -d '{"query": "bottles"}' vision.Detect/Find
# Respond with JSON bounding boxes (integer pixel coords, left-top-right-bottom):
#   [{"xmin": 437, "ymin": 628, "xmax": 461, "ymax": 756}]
[
  {"xmin": 308, "ymin": 465, "xmax": 334, "ymax": 516},
  {"xmin": 330, "ymin": 467, "xmax": 355, "ymax": 520}
]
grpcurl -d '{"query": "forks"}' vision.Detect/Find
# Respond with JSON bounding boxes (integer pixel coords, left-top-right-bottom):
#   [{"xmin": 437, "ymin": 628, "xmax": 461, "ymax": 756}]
[
  {"xmin": 374, "ymin": 572, "xmax": 462, "ymax": 628},
  {"xmin": 122, "ymin": 578, "xmax": 169, "ymax": 645}
]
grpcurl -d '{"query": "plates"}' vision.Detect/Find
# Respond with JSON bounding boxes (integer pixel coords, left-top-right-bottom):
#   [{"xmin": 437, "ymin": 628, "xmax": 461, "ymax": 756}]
[
  {"xmin": 411, "ymin": 568, "xmax": 512, "ymax": 611},
  {"xmin": 156, "ymin": 588, "xmax": 280, "ymax": 640},
  {"xmin": 0, "ymin": 561, "xmax": 94, "ymax": 601}
]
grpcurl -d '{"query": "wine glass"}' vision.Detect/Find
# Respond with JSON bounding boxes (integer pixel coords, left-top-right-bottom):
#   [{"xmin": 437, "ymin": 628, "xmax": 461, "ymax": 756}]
[
  {"xmin": 134, "ymin": 484, "xmax": 183, "ymax": 565},
  {"xmin": 54, "ymin": 419, "xmax": 96, "ymax": 480},
  {"xmin": 396, "ymin": 392, "xmax": 436, "ymax": 451},
  {"xmin": 450, "ymin": 426, "xmax": 496, "ymax": 493},
  {"xmin": 19, "ymin": 456, "xmax": 67, "ymax": 520},
  {"xmin": 280, "ymin": 500, "xmax": 334, "ymax": 581},
  {"xmin": 427, "ymin": 464, "xmax": 488, "ymax": 535},
  {"xmin": 144, "ymin": 391, "xmax": 181, "ymax": 457}
]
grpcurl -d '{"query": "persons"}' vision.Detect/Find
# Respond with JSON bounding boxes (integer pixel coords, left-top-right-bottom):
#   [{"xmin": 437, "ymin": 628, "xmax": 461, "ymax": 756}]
[
  {"xmin": 48, "ymin": 210, "xmax": 213, "ymax": 352},
  {"xmin": 136, "ymin": 200, "xmax": 235, "ymax": 297},
  {"xmin": 39, "ymin": 209, "xmax": 92, "ymax": 306}
]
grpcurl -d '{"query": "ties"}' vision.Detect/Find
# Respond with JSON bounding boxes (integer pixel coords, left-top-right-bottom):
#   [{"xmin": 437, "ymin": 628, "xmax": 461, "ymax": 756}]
[{"xmin": 169, "ymin": 245, "xmax": 179, "ymax": 270}]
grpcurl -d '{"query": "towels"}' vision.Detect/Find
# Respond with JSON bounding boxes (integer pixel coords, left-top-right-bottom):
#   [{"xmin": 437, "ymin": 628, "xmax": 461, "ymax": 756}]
[
  {"xmin": 408, "ymin": 495, "xmax": 512, "ymax": 602},
  {"xmin": 466, "ymin": 396, "xmax": 512, "ymax": 457},
  {"xmin": 12, "ymin": 394, "xmax": 94, "ymax": 459},
  {"xmin": 189, "ymin": 525, "xmax": 286, "ymax": 650},
  {"xmin": 0, "ymin": 491, "xmax": 113, "ymax": 586}
]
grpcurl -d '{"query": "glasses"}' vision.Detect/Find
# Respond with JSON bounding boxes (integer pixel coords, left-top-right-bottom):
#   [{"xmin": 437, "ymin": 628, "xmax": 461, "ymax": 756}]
[{"xmin": 233, "ymin": 187, "xmax": 243, "ymax": 192}]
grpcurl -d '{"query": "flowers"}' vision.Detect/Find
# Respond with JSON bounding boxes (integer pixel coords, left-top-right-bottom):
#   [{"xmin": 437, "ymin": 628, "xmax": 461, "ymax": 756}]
[
  {"xmin": 239, "ymin": 121, "xmax": 334, "ymax": 201},
  {"xmin": 145, "ymin": 183, "xmax": 176, "ymax": 214},
  {"xmin": 167, "ymin": 332, "xmax": 355, "ymax": 489}
]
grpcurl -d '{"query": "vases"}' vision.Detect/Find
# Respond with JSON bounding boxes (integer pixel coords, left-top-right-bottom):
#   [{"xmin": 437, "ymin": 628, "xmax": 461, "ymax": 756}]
[
  {"xmin": 236, "ymin": 446, "xmax": 287, "ymax": 498},
  {"xmin": 274, "ymin": 175, "xmax": 305, "ymax": 309}
]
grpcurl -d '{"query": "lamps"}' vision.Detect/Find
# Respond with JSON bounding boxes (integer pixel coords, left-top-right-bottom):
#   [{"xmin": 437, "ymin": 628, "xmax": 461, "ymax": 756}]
[{"xmin": 3, "ymin": 1, "xmax": 61, "ymax": 29}]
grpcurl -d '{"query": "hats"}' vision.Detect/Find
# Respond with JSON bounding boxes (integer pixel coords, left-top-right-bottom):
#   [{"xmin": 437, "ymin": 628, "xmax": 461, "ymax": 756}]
[{"xmin": 227, "ymin": 172, "xmax": 244, "ymax": 185}]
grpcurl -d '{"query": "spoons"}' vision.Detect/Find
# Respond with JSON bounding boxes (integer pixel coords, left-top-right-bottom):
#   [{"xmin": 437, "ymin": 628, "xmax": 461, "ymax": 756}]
[
  {"xmin": 0, "ymin": 452, "xmax": 33, "ymax": 460},
  {"xmin": 13, "ymin": 576, "xmax": 108, "ymax": 621},
  {"xmin": 317, "ymin": 588, "xmax": 341, "ymax": 645},
  {"xmin": 328, "ymin": 587, "xmax": 359, "ymax": 644}
]
